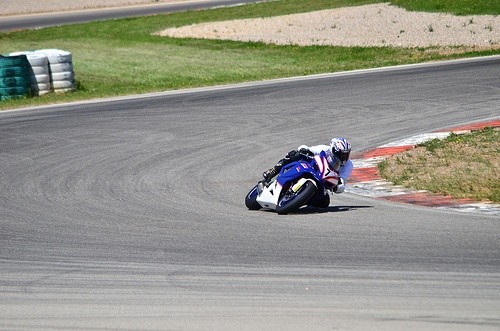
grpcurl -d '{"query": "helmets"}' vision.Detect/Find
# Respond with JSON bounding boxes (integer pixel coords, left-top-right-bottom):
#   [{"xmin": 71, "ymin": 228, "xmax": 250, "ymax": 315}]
[{"xmin": 331, "ymin": 137, "xmax": 351, "ymax": 164}]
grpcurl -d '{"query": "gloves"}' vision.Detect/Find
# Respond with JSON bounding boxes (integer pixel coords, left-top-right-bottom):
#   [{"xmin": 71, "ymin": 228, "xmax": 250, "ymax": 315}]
[{"xmin": 299, "ymin": 148, "xmax": 309, "ymax": 156}]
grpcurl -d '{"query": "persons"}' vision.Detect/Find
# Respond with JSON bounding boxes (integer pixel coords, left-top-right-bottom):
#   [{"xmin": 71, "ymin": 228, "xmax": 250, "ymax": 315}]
[{"xmin": 262, "ymin": 137, "xmax": 353, "ymax": 208}]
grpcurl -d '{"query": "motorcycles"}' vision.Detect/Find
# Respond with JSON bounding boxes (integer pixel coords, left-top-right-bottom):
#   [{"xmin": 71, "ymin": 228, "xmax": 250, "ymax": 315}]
[{"xmin": 245, "ymin": 148, "xmax": 343, "ymax": 215}]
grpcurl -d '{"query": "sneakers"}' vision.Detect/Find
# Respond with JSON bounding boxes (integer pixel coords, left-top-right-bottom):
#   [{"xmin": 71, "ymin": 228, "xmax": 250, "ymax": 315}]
[{"xmin": 263, "ymin": 167, "xmax": 276, "ymax": 179}]
